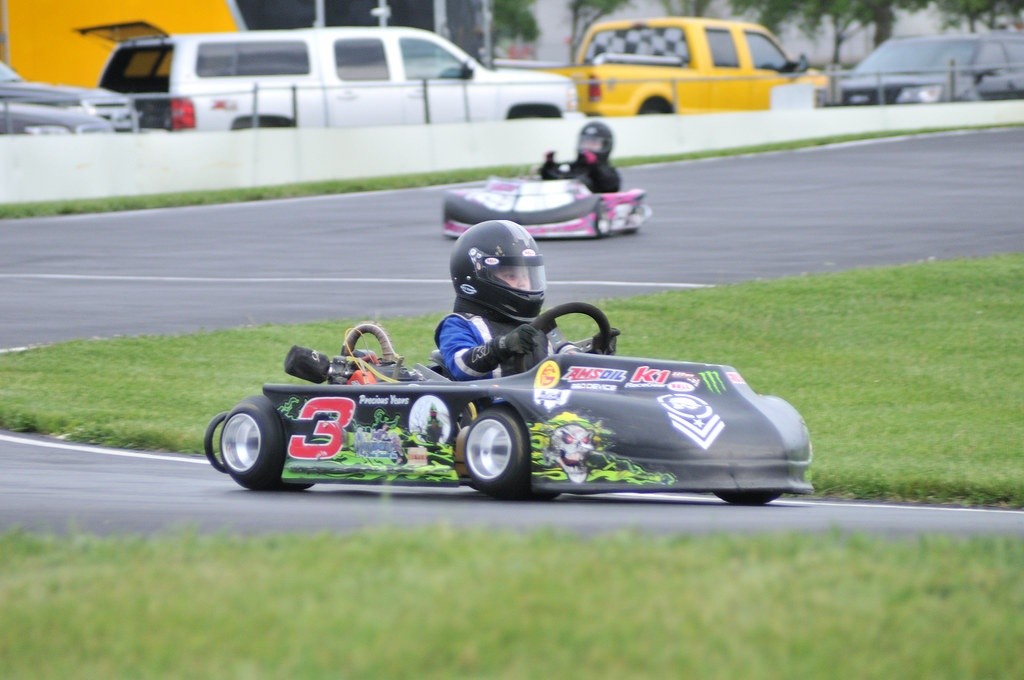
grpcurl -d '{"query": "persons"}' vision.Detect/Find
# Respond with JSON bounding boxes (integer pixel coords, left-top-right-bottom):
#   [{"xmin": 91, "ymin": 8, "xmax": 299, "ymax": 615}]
[
  {"xmin": 434, "ymin": 220, "xmax": 619, "ymax": 381},
  {"xmin": 539, "ymin": 121, "xmax": 619, "ymax": 192}
]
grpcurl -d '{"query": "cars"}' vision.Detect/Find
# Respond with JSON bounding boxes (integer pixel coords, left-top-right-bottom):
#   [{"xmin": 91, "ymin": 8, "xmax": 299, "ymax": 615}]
[
  {"xmin": 0, "ymin": 60, "xmax": 139, "ymax": 134},
  {"xmin": 0, "ymin": 100, "xmax": 113, "ymax": 133},
  {"xmin": 841, "ymin": 30, "xmax": 1024, "ymax": 102}
]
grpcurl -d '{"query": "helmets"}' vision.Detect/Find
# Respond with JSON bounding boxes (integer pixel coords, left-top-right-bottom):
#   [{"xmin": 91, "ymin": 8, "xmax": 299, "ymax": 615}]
[
  {"xmin": 577, "ymin": 122, "xmax": 613, "ymax": 160},
  {"xmin": 449, "ymin": 220, "xmax": 547, "ymax": 326}
]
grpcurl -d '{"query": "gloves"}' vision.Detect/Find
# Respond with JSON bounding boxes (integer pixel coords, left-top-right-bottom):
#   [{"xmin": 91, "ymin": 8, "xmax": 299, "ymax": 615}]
[
  {"xmin": 493, "ymin": 323, "xmax": 539, "ymax": 359},
  {"xmin": 541, "ymin": 161, "xmax": 559, "ymax": 170},
  {"xmin": 592, "ymin": 328, "xmax": 620, "ymax": 356}
]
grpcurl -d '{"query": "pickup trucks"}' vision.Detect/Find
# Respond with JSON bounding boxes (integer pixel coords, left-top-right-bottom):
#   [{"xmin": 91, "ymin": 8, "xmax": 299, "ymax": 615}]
[
  {"xmin": 496, "ymin": 17, "xmax": 828, "ymax": 116},
  {"xmin": 74, "ymin": 21, "xmax": 577, "ymax": 134}
]
